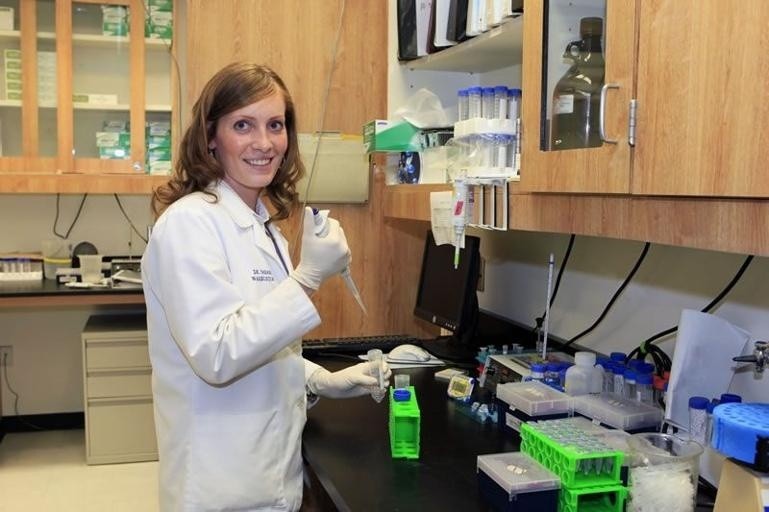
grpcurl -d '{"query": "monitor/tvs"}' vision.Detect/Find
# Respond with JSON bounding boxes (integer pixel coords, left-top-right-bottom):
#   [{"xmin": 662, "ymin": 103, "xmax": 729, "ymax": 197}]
[{"xmin": 414, "ymin": 230, "xmax": 481, "ymax": 365}]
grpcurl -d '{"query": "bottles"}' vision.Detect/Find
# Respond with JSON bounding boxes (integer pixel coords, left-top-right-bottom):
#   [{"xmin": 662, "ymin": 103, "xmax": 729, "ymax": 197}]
[
  {"xmin": 522, "ymin": 350, "xmax": 670, "ymax": 410},
  {"xmin": 394, "ymin": 374, "xmax": 411, "ymax": 392},
  {"xmin": 477, "ymin": 344, "xmax": 525, "ymax": 355},
  {"xmin": 367, "ymin": 347, "xmax": 387, "ymax": 403},
  {"xmin": 1, "ymin": 257, "xmax": 43, "ymax": 275},
  {"xmin": 544, "ymin": 14, "xmax": 609, "ymax": 152},
  {"xmin": 521, "ymin": 417, "xmax": 620, "ymax": 477},
  {"xmin": 688, "ymin": 390, "xmax": 743, "ymax": 446},
  {"xmin": 455, "ymin": 85, "xmax": 522, "ymax": 172}
]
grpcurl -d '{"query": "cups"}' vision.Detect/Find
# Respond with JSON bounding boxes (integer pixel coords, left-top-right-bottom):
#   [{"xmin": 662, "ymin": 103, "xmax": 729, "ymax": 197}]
[
  {"xmin": 42, "ymin": 240, "xmax": 73, "ymax": 281},
  {"xmin": 77, "ymin": 254, "xmax": 106, "ymax": 285},
  {"xmin": 624, "ymin": 429, "xmax": 705, "ymax": 512}
]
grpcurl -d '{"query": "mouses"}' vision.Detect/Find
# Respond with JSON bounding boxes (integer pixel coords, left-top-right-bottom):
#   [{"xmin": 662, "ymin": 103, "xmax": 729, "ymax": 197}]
[{"xmin": 388, "ymin": 344, "xmax": 431, "ymax": 362}]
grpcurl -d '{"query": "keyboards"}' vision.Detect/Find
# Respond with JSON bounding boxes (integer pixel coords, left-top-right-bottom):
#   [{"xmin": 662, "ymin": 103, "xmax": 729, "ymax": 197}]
[{"xmin": 302, "ymin": 334, "xmax": 422, "ymax": 354}]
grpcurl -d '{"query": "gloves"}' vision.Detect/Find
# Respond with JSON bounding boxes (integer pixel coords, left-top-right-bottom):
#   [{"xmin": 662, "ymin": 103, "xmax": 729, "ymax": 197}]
[
  {"xmin": 308, "ymin": 360, "xmax": 393, "ymax": 400},
  {"xmin": 291, "ymin": 207, "xmax": 352, "ymax": 291}
]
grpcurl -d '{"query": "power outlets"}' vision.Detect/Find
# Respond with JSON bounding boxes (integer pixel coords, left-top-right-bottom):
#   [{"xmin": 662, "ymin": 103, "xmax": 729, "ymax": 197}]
[{"xmin": 0, "ymin": 346, "xmax": 13, "ymax": 365}]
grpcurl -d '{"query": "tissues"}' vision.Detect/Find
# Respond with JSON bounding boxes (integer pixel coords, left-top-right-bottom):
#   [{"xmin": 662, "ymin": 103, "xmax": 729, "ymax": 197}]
[{"xmin": 362, "ymin": 88, "xmax": 457, "ymax": 154}]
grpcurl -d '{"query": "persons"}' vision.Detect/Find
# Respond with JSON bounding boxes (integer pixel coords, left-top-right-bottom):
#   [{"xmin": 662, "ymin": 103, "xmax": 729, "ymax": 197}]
[{"xmin": 139, "ymin": 61, "xmax": 393, "ymax": 512}]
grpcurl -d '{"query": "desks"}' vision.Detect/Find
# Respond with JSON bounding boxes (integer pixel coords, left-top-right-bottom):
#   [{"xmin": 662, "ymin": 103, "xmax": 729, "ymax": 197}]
[{"xmin": 301, "ymin": 334, "xmax": 718, "ymax": 512}]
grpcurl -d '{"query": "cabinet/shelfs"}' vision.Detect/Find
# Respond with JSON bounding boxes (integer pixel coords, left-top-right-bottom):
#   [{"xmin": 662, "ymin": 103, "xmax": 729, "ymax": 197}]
[
  {"xmin": 81, "ymin": 314, "xmax": 159, "ymax": 465},
  {"xmin": 519, "ymin": 0, "xmax": 769, "ymax": 198},
  {"xmin": 0, "ymin": 1, "xmax": 181, "ymax": 195},
  {"xmin": 387, "ymin": 1, "xmax": 522, "ymax": 184}
]
